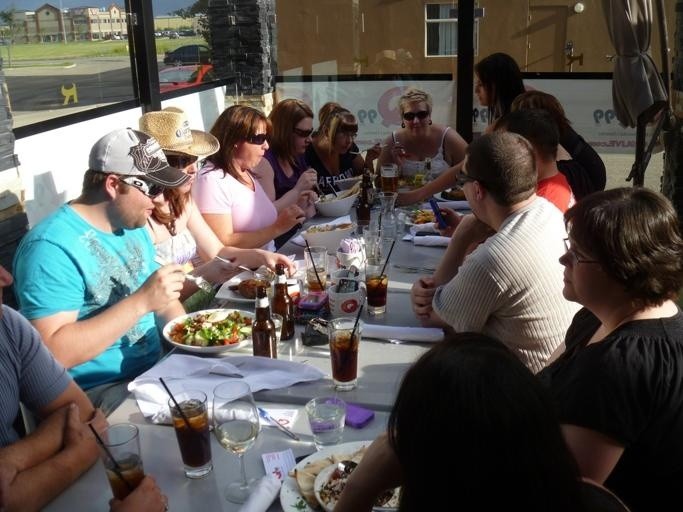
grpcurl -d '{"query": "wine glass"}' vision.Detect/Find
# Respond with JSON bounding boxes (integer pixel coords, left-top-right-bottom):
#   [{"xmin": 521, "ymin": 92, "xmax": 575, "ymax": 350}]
[{"xmin": 212, "ymin": 381, "xmax": 260, "ymax": 503}]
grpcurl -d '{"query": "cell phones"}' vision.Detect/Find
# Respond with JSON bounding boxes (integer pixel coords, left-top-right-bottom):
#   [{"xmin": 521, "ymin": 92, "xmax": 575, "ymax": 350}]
[
  {"xmin": 326, "ymin": 399, "xmax": 375, "ymax": 429},
  {"xmin": 430, "ymin": 198, "xmax": 447, "ymax": 228}
]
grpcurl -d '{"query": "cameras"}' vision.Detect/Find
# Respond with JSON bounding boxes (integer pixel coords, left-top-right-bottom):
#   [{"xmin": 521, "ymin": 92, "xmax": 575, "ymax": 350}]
[{"xmin": 297, "ymin": 291, "xmax": 328, "ymax": 311}]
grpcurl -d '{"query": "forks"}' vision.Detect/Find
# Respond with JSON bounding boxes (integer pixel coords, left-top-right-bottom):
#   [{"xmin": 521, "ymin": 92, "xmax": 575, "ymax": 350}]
[{"xmin": 151, "ymin": 257, "xmax": 212, "ymax": 294}]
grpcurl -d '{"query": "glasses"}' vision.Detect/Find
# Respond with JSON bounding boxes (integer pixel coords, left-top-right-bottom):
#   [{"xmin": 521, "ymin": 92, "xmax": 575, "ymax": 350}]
[
  {"xmin": 293, "ymin": 127, "xmax": 313, "ymax": 138},
  {"xmin": 120, "ymin": 175, "xmax": 166, "ymax": 198},
  {"xmin": 336, "ymin": 131, "xmax": 358, "ymax": 140},
  {"xmin": 454, "ymin": 170, "xmax": 477, "ymax": 186},
  {"xmin": 246, "ymin": 133, "xmax": 270, "ymax": 144},
  {"xmin": 168, "ymin": 155, "xmax": 197, "ymax": 169},
  {"xmin": 402, "ymin": 110, "xmax": 428, "ymax": 121},
  {"xmin": 562, "ymin": 238, "xmax": 598, "ymax": 267}
]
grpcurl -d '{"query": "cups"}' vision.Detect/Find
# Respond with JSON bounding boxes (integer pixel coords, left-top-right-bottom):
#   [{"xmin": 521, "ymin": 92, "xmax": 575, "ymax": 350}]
[
  {"xmin": 305, "ymin": 396, "xmax": 347, "ymax": 451},
  {"xmin": 95, "ymin": 422, "xmax": 144, "ymax": 500},
  {"xmin": 167, "ymin": 390, "xmax": 215, "ymax": 480},
  {"xmin": 327, "ymin": 317, "xmax": 360, "ymax": 390}
]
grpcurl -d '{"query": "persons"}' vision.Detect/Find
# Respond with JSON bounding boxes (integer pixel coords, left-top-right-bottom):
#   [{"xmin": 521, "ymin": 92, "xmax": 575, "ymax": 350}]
[
  {"xmin": 190, "ymin": 105, "xmax": 323, "ymax": 271},
  {"xmin": 247, "ymin": 99, "xmax": 320, "ymax": 251},
  {"xmin": 534, "ymin": 182, "xmax": 681, "ymax": 510},
  {"xmin": 302, "ymin": 101, "xmax": 384, "ymax": 199},
  {"xmin": 135, "ymin": 105, "xmax": 299, "ymax": 316},
  {"xmin": 393, "ymin": 48, "xmax": 609, "ymax": 376},
  {"xmin": 0, "ymin": 261, "xmax": 107, "ymax": 511},
  {"xmin": 331, "ymin": 329, "xmax": 636, "ymax": 512},
  {"xmin": 10, "ymin": 127, "xmax": 196, "ymax": 420},
  {"xmin": 103, "ymin": 471, "xmax": 169, "ymax": 511},
  {"xmin": 373, "ymin": 87, "xmax": 469, "ymax": 183}
]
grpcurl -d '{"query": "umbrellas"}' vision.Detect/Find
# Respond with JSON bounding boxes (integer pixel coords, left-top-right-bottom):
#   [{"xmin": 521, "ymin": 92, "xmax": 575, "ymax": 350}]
[{"xmin": 598, "ymin": 1, "xmax": 668, "ymax": 187}]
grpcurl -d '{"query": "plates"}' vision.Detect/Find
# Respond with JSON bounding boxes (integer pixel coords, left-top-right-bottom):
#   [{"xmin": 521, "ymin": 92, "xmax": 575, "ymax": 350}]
[
  {"xmin": 161, "ymin": 308, "xmax": 255, "ymax": 353},
  {"xmin": 313, "ymin": 462, "xmax": 339, "ymax": 512},
  {"xmin": 279, "ymin": 440, "xmax": 401, "ymax": 512},
  {"xmin": 213, "ymin": 271, "xmax": 263, "ymax": 305}
]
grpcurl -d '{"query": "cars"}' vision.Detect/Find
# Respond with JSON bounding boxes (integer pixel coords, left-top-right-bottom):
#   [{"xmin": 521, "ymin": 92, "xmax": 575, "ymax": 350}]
[
  {"xmin": 157, "ymin": 65, "xmax": 215, "ymax": 95},
  {"xmin": 110, "ymin": 29, "xmax": 195, "ymax": 41},
  {"xmin": 161, "ymin": 44, "xmax": 215, "ymax": 68}
]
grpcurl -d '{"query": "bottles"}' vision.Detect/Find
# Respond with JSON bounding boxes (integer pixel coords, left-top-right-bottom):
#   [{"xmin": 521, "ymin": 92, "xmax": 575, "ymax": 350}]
[{"xmin": 250, "ymin": 159, "xmax": 466, "ymax": 359}]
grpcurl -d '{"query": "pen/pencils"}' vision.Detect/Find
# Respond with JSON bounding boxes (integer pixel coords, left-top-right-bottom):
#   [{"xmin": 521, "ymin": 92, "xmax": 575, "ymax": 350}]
[{"xmin": 257, "ymin": 406, "xmax": 300, "ymax": 441}]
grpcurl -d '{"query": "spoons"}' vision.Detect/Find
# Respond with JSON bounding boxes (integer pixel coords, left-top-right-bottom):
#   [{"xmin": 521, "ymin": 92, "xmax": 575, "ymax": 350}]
[{"xmin": 337, "ymin": 459, "xmax": 357, "ymax": 476}]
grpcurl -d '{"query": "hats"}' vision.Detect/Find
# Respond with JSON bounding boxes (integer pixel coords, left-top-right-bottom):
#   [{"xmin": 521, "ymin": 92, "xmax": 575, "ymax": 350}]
[
  {"xmin": 89, "ymin": 127, "xmax": 191, "ymax": 189},
  {"xmin": 138, "ymin": 106, "xmax": 220, "ymax": 159}
]
